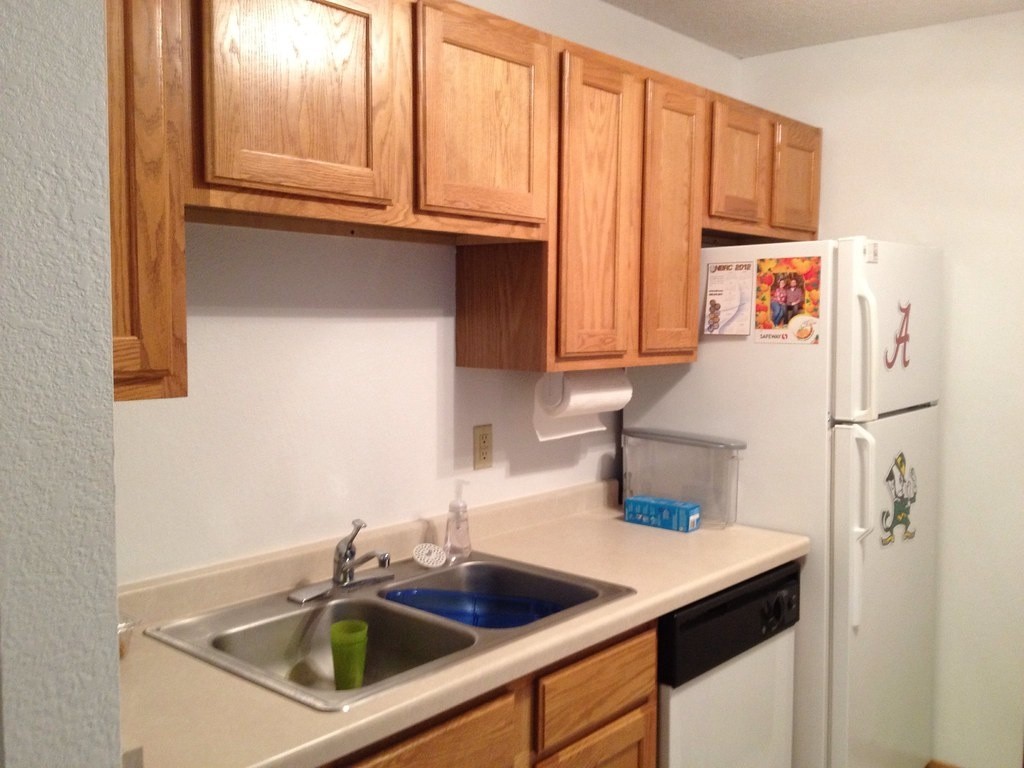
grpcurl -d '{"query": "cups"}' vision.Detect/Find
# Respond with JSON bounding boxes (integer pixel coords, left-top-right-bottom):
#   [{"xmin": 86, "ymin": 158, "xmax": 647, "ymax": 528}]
[{"xmin": 327, "ymin": 617, "xmax": 368, "ymax": 692}]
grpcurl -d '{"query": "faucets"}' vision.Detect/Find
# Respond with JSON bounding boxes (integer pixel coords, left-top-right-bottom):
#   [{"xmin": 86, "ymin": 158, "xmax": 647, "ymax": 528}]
[{"xmin": 332, "ymin": 518, "xmax": 390, "ymax": 586}]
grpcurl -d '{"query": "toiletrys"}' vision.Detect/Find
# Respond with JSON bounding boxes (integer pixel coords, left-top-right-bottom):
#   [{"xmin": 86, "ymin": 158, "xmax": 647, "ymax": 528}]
[{"xmin": 442, "ymin": 478, "xmax": 473, "ymax": 562}]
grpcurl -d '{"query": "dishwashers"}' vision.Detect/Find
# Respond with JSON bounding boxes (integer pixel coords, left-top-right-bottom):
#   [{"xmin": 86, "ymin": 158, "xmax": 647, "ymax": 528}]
[{"xmin": 656, "ymin": 559, "xmax": 801, "ymax": 768}]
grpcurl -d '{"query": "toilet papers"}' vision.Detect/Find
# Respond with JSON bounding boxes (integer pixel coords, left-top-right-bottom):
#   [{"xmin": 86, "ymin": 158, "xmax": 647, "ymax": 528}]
[{"xmin": 530, "ymin": 372, "xmax": 633, "ymax": 442}]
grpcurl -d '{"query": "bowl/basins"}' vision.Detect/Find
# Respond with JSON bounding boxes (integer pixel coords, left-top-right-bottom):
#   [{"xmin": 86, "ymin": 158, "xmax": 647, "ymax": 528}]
[{"xmin": 385, "ymin": 587, "xmax": 565, "ymax": 630}]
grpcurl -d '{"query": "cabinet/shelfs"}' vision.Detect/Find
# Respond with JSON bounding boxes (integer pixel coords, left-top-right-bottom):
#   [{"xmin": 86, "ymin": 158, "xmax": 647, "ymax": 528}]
[
  {"xmin": 185, "ymin": 0, "xmax": 551, "ymax": 248},
  {"xmin": 454, "ymin": 35, "xmax": 702, "ymax": 373},
  {"xmin": 320, "ymin": 618, "xmax": 658, "ymax": 768},
  {"xmin": 702, "ymin": 88, "xmax": 823, "ymax": 242},
  {"xmin": 105, "ymin": 0, "xmax": 189, "ymax": 403}
]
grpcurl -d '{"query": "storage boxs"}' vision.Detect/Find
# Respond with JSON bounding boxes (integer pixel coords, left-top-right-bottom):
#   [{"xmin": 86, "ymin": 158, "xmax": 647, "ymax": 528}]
[{"xmin": 623, "ymin": 495, "xmax": 700, "ymax": 533}]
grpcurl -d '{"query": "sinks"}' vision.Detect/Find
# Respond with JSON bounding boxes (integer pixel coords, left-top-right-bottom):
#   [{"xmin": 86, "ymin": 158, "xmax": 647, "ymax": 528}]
[
  {"xmin": 139, "ymin": 582, "xmax": 478, "ymax": 713},
  {"xmin": 370, "ymin": 541, "xmax": 638, "ymax": 644}
]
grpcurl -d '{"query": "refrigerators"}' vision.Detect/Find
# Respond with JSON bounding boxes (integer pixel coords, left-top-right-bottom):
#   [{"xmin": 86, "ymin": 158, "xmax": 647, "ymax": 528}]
[{"xmin": 618, "ymin": 233, "xmax": 946, "ymax": 768}]
